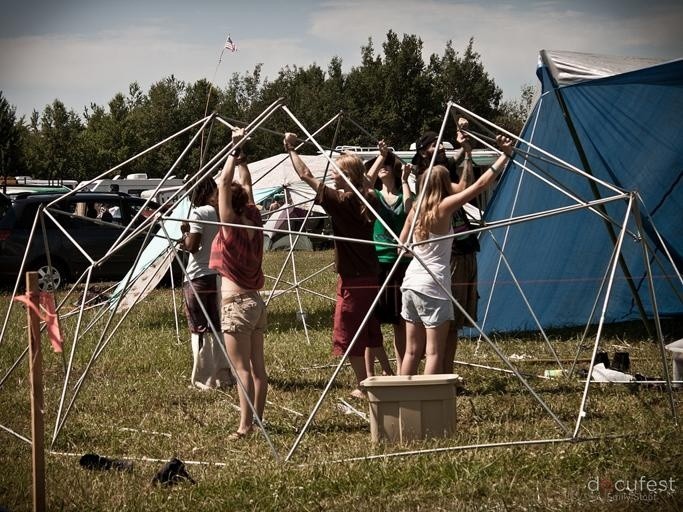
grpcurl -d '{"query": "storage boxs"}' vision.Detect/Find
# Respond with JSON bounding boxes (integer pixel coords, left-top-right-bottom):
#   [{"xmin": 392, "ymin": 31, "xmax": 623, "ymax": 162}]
[
  {"xmin": 664, "ymin": 338, "xmax": 683, "ymax": 387},
  {"xmin": 360, "ymin": 373, "xmax": 463, "ymax": 447}
]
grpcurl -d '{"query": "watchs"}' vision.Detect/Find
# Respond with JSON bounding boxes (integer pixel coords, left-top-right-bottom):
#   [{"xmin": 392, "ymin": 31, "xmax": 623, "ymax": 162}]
[{"xmin": 229, "ymin": 147, "xmax": 240, "ymax": 159}]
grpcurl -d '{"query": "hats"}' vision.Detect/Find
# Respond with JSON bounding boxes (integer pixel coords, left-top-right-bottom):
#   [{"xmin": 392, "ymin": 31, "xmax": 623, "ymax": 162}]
[{"xmin": 410, "ymin": 132, "xmax": 443, "ymax": 166}]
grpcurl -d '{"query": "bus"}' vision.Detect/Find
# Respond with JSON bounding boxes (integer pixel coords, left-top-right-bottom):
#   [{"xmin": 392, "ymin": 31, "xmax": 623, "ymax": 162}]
[{"xmin": 334, "ymin": 145, "xmax": 504, "ymax": 211}]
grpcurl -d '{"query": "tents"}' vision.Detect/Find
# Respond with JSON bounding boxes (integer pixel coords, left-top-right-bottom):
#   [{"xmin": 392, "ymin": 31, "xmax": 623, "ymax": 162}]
[{"xmin": 456, "ymin": 48, "xmax": 682, "ymax": 342}]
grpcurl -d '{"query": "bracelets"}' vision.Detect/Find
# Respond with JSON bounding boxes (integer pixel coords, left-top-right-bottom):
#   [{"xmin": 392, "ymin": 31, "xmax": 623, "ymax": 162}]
[
  {"xmin": 488, "ymin": 165, "xmax": 500, "ymax": 177},
  {"xmin": 181, "ymin": 231, "xmax": 190, "ymax": 240},
  {"xmin": 462, "ymin": 156, "xmax": 473, "ymax": 161},
  {"xmin": 282, "ymin": 142, "xmax": 295, "ymax": 153},
  {"xmin": 401, "ymin": 181, "xmax": 408, "ymax": 184}
]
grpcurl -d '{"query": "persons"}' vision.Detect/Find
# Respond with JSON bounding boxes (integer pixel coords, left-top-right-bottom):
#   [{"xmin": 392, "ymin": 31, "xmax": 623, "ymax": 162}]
[
  {"xmin": 359, "ymin": 138, "xmax": 418, "ymax": 376},
  {"xmin": 280, "ymin": 129, "xmax": 393, "ymax": 405},
  {"xmin": 207, "ymin": 125, "xmax": 269, "ymax": 444},
  {"xmin": 71, "ymin": 183, "xmax": 125, "ymax": 225},
  {"xmin": 267, "ymin": 195, "xmax": 281, "ymax": 210},
  {"xmin": 396, "ymin": 134, "xmax": 514, "ymax": 377},
  {"xmin": 410, "ymin": 115, "xmax": 481, "ymax": 397},
  {"xmin": 179, "ymin": 170, "xmax": 236, "ymax": 390}
]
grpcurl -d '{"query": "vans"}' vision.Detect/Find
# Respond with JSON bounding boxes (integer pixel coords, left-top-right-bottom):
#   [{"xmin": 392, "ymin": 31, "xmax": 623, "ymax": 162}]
[{"xmin": 0, "ymin": 172, "xmax": 189, "ymax": 294}]
